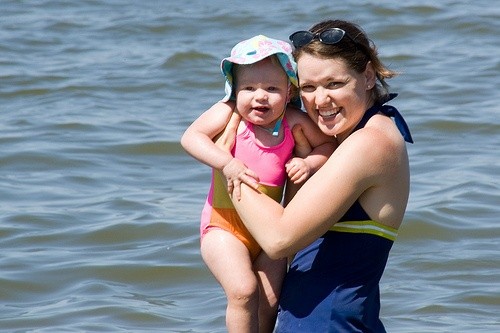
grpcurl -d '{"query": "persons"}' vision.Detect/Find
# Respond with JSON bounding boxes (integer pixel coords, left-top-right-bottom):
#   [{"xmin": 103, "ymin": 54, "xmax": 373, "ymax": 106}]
[
  {"xmin": 212, "ymin": 18, "xmax": 413, "ymax": 333},
  {"xmin": 179, "ymin": 34, "xmax": 335, "ymax": 333}
]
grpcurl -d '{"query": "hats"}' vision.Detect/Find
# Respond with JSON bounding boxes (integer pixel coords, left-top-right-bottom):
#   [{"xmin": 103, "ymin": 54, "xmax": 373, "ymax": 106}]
[{"xmin": 220, "ymin": 35, "xmax": 299, "ymax": 104}]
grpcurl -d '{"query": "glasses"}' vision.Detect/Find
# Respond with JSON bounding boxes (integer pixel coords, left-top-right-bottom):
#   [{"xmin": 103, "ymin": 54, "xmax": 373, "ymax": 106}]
[{"xmin": 290, "ymin": 29, "xmax": 368, "ymax": 53}]
